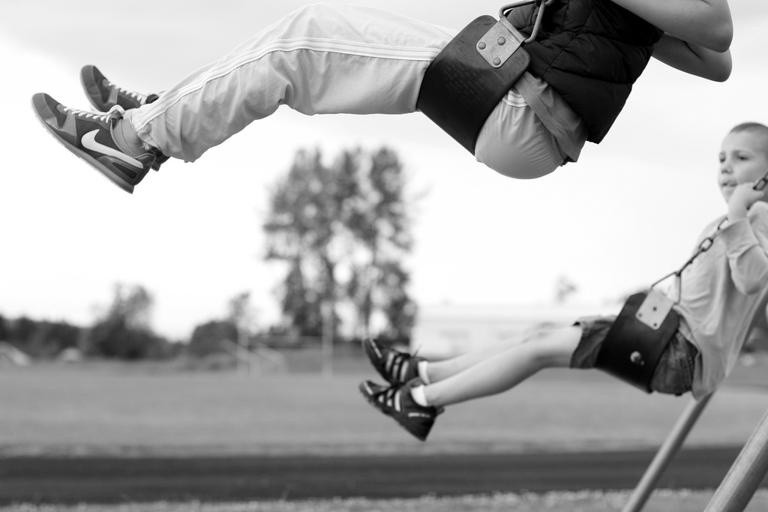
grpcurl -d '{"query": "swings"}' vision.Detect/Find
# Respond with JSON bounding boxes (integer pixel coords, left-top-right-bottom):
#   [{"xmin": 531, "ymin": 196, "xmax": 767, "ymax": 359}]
[
  {"xmin": 597, "ymin": 176, "xmax": 766, "ymax": 396},
  {"xmin": 414, "ymin": 0, "xmax": 544, "ymax": 158}
]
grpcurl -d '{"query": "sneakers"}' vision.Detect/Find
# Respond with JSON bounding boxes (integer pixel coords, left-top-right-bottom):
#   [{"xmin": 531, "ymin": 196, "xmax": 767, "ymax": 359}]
[
  {"xmin": 33, "ymin": 65, "xmax": 170, "ymax": 193},
  {"xmin": 359, "ymin": 338, "xmax": 436, "ymax": 441}
]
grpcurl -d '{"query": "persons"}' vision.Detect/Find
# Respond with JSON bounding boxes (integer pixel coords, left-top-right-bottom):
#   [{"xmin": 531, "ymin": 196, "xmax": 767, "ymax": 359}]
[
  {"xmin": 359, "ymin": 121, "xmax": 768, "ymax": 440},
  {"xmin": 31, "ymin": 0, "xmax": 735, "ymax": 196}
]
